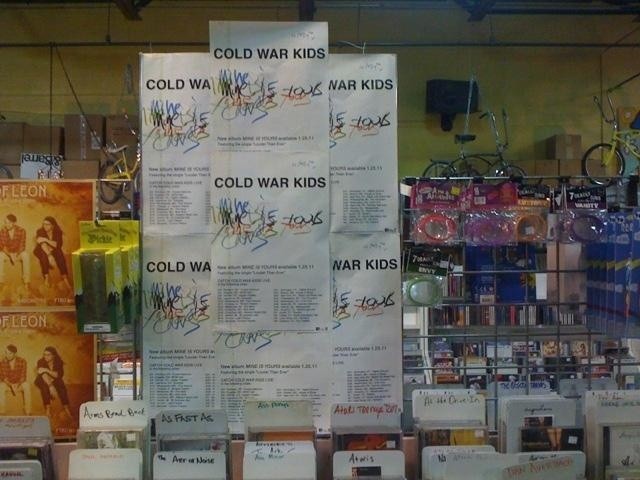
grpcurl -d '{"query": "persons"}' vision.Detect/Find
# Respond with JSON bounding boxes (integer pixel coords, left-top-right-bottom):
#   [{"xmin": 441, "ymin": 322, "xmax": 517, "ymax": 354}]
[
  {"xmin": 34, "ymin": 344, "xmax": 78, "ymax": 421},
  {"xmin": 31, "ymin": 215, "xmax": 77, "ymax": 291},
  {"xmin": 0, "ymin": 345, "xmax": 34, "ymax": 418},
  {"xmin": 1, "ymin": 213, "xmax": 32, "ymax": 290}
]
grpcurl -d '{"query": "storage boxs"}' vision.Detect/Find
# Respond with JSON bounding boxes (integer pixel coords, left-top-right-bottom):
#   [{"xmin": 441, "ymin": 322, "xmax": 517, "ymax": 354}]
[
  {"xmin": 509, "ymin": 133, "xmax": 606, "ymax": 189},
  {"xmin": 0, "ymin": 112, "xmax": 139, "ymax": 213}
]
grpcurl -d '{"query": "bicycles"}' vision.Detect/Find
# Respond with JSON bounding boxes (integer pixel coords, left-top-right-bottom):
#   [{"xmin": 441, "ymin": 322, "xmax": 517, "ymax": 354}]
[
  {"xmin": 421, "ymin": 110, "xmax": 527, "ymax": 186},
  {"xmin": 96, "ymin": 123, "xmax": 140, "ymax": 204},
  {"xmin": 581, "ymin": 86, "xmax": 640, "ymax": 187}
]
